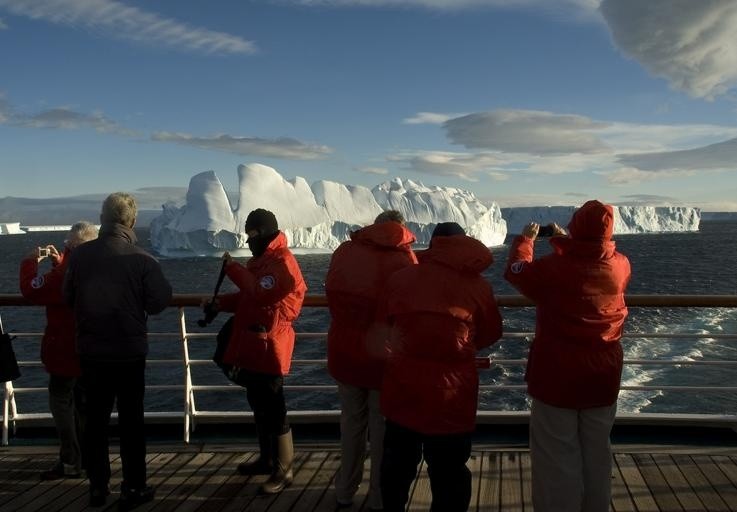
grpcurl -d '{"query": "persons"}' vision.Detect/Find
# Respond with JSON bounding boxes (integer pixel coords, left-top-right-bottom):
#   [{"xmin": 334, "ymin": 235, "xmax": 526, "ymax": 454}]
[
  {"xmin": 503, "ymin": 199, "xmax": 631, "ymax": 511},
  {"xmin": 64, "ymin": 190, "xmax": 172, "ymax": 505},
  {"xmin": 358, "ymin": 221, "xmax": 503, "ymax": 512},
  {"xmin": 19, "ymin": 220, "xmax": 99, "ymax": 480},
  {"xmin": 324, "ymin": 210, "xmax": 419, "ymax": 512},
  {"xmin": 200, "ymin": 208, "xmax": 308, "ymax": 493}
]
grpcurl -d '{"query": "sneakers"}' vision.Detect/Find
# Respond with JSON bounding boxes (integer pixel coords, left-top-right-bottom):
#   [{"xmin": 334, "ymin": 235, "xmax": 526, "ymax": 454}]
[{"xmin": 89, "ymin": 481, "xmax": 156, "ymax": 509}]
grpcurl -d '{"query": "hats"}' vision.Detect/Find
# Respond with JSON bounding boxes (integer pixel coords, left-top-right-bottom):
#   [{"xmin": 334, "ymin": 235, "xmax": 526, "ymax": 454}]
[{"xmin": 245, "ymin": 208, "xmax": 278, "ymax": 235}]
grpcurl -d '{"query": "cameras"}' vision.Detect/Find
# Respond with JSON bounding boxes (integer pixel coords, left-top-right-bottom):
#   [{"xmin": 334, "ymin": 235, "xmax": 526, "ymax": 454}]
[
  {"xmin": 35, "ymin": 248, "xmax": 50, "ymax": 257},
  {"xmin": 537, "ymin": 226, "xmax": 552, "ymax": 237}
]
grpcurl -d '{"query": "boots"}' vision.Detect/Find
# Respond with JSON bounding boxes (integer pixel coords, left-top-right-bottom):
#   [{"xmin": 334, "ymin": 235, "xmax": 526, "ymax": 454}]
[{"xmin": 237, "ymin": 428, "xmax": 294, "ymax": 495}]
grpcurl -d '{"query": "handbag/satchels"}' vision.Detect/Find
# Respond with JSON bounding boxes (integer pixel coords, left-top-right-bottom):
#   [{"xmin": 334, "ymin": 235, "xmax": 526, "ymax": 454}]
[{"xmin": 212, "ymin": 314, "xmax": 268, "ymax": 387}]
